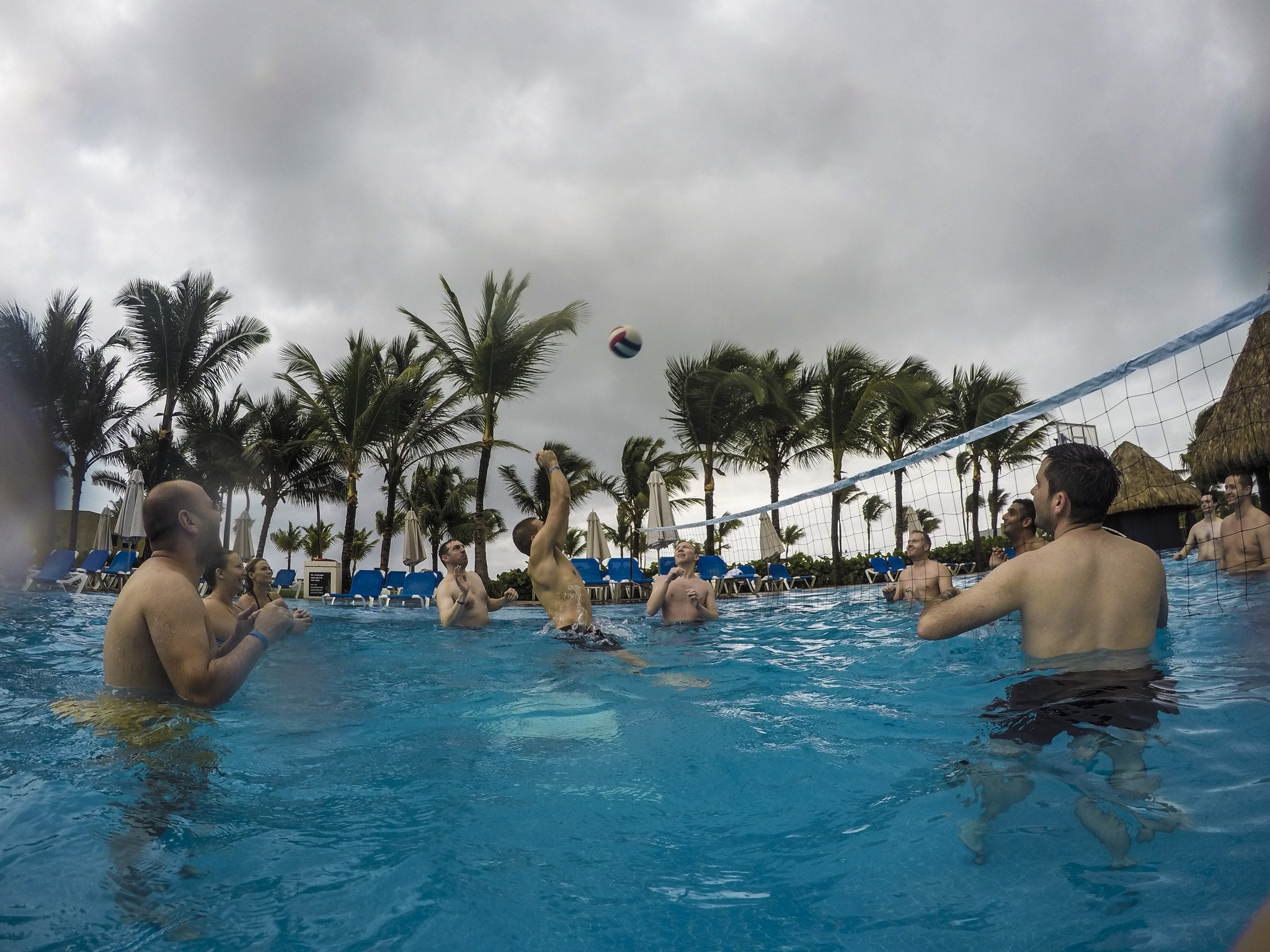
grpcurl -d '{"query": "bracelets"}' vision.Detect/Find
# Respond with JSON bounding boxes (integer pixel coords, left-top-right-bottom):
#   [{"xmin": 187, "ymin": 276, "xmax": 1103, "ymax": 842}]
[
  {"xmin": 549, "ymin": 466, "xmax": 560, "ymax": 474},
  {"xmin": 249, "ymin": 630, "xmax": 270, "ymax": 650},
  {"xmin": 457, "ymin": 599, "xmax": 465, "ymax": 605},
  {"xmin": 664, "ymin": 577, "xmax": 671, "ymax": 584}
]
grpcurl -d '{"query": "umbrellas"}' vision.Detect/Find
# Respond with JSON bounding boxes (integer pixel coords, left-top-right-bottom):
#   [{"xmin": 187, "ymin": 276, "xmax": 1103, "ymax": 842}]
[
  {"xmin": 907, "ymin": 504, "xmax": 923, "ymax": 535},
  {"xmin": 586, "ymin": 508, "xmax": 612, "ymax": 570},
  {"xmin": 759, "ymin": 512, "xmax": 785, "ymax": 578},
  {"xmin": 647, "ymin": 466, "xmax": 680, "ymax": 576},
  {"xmin": 404, "ymin": 510, "xmax": 427, "ymax": 573},
  {"xmin": 94, "ymin": 468, "xmax": 256, "ymax": 571}
]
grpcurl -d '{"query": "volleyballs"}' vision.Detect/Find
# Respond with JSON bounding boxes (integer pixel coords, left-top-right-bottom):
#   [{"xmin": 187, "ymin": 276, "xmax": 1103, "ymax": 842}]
[{"xmin": 608, "ymin": 326, "xmax": 643, "ymax": 359}]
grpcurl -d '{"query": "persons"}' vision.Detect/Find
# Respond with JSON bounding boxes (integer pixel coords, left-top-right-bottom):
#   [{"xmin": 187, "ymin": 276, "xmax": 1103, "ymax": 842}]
[
  {"xmin": 1173, "ymin": 490, "xmax": 1226, "ymax": 563},
  {"xmin": 646, "ymin": 539, "xmax": 719, "ymax": 622},
  {"xmin": 101, "ymin": 481, "xmax": 314, "ymax": 705},
  {"xmin": 1220, "ymin": 469, "xmax": 1270, "ymax": 570},
  {"xmin": 883, "ymin": 443, "xmax": 1168, "ymax": 657},
  {"xmin": 438, "ymin": 538, "xmax": 519, "ymax": 627},
  {"xmin": 512, "ymin": 450, "xmax": 594, "ymax": 632}
]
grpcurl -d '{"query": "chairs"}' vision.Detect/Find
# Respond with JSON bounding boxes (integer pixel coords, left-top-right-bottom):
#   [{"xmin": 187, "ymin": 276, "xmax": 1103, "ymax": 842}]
[
  {"xmin": 570, "ymin": 555, "xmax": 816, "ymax": 602},
  {"xmin": 864, "ymin": 546, "xmax": 1016, "ymax": 584},
  {"xmin": 21, "ymin": 549, "xmax": 296, "ymax": 599},
  {"xmin": 320, "ymin": 568, "xmax": 444, "ymax": 610}
]
